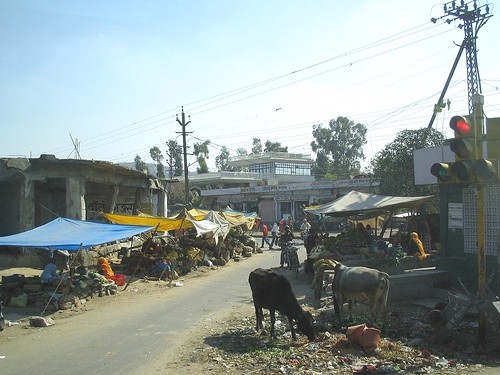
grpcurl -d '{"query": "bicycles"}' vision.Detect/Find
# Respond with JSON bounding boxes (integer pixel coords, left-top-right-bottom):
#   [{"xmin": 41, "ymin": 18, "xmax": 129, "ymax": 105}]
[{"xmin": 290, "ymin": 247, "xmax": 299, "ymax": 279}]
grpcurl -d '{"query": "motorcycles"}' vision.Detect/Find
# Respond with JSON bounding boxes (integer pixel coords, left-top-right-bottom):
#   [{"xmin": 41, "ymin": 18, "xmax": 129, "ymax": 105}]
[{"xmin": 278, "ymin": 241, "xmax": 295, "ymax": 270}]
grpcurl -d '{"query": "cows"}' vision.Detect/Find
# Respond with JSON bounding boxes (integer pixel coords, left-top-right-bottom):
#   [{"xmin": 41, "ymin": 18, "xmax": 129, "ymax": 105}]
[
  {"xmin": 333, "ymin": 263, "xmax": 390, "ymax": 329},
  {"xmin": 249, "ymin": 268, "xmax": 316, "ymax": 342}
]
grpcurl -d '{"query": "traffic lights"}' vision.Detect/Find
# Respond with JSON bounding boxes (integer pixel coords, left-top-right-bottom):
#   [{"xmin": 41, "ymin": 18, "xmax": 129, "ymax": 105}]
[
  {"xmin": 448, "ymin": 114, "xmax": 475, "ymax": 160},
  {"xmin": 430, "ymin": 160, "xmax": 497, "ymax": 185}
]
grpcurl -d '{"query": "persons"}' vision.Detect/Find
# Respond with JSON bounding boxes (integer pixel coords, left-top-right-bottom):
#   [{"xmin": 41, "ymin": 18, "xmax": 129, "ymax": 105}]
[
  {"xmin": 301, "ymin": 218, "xmax": 312, "ymax": 247},
  {"xmin": 406, "ymin": 232, "xmax": 425, "ymax": 257},
  {"xmin": 260, "ymin": 222, "xmax": 271, "ymax": 248},
  {"xmin": 287, "ymin": 215, "xmax": 294, "ymax": 229},
  {"xmin": 41, "ymin": 259, "xmax": 61, "ymax": 283},
  {"xmin": 280, "ymin": 226, "xmax": 302, "ymax": 269},
  {"xmin": 357, "ymin": 222, "xmax": 374, "ymax": 238},
  {"xmin": 269, "ymin": 222, "xmax": 279, "ymax": 250}
]
